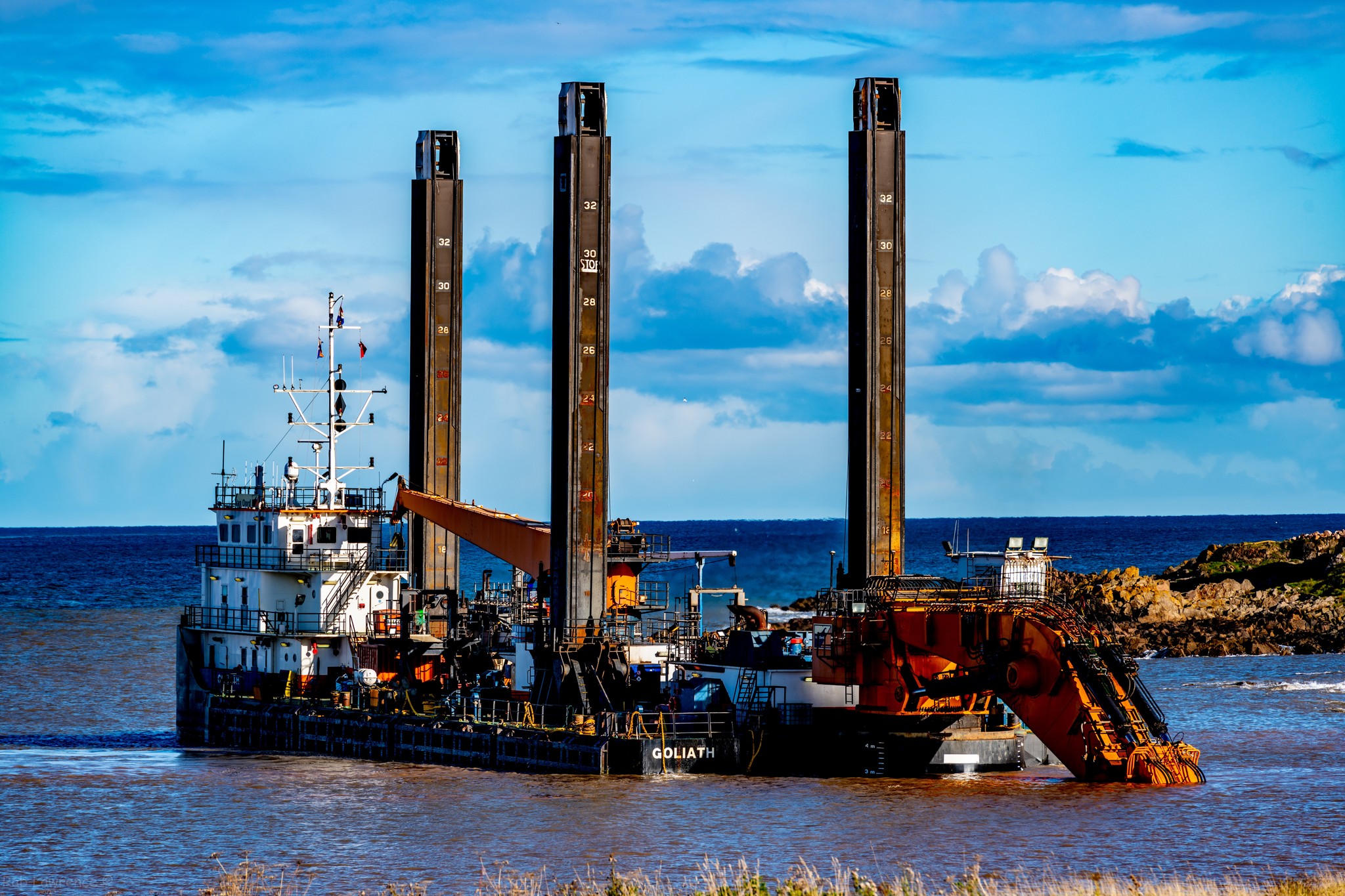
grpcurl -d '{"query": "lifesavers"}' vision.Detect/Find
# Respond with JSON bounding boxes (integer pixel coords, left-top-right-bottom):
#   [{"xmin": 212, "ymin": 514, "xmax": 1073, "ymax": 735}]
[{"xmin": 668, "ymin": 696, "xmax": 677, "ymax": 713}]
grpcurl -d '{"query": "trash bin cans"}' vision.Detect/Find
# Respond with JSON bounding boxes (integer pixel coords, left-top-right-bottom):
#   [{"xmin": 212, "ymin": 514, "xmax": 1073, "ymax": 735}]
[{"xmin": 678, "ymin": 677, "xmax": 711, "ymax": 715}]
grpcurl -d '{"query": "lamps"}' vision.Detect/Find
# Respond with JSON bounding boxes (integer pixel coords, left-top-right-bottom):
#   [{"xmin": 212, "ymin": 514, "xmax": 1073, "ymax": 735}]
[
  {"xmin": 291, "ymin": 516, "xmax": 307, "ymax": 521},
  {"xmin": 297, "ymin": 579, "xmax": 305, "ymax": 583},
  {"xmin": 218, "ymin": 501, "xmax": 222, "ymax": 508},
  {"xmin": 691, "ymin": 672, "xmax": 701, "ymax": 677},
  {"xmin": 740, "ymin": 675, "xmax": 750, "ymax": 680},
  {"xmin": 253, "ymin": 516, "xmax": 264, "ymax": 521},
  {"xmin": 280, "ymin": 642, "xmax": 290, "ymax": 647},
  {"xmin": 376, "ymin": 504, "xmax": 379, "ymax": 510},
  {"xmin": 209, "ymin": 575, "xmax": 219, "ymax": 580},
  {"xmin": 323, "ymin": 581, "xmax": 338, "ymax": 584},
  {"xmin": 234, "ymin": 577, "xmax": 244, "ymax": 582},
  {"xmin": 223, "ymin": 514, "xmax": 233, "ymax": 519},
  {"xmin": 317, "ymin": 644, "xmax": 331, "ymax": 648},
  {"xmin": 803, "ymin": 677, "xmax": 812, "ymax": 681},
  {"xmin": 658, "ymin": 652, "xmax": 674, "ymax": 656},
  {"xmin": 212, "ymin": 636, "xmax": 225, "ymax": 644},
  {"xmin": 250, "ymin": 639, "xmax": 256, "ymax": 644}
]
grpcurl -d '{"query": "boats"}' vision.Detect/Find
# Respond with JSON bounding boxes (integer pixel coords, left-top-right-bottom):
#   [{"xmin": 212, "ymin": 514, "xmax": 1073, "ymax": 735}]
[
  {"xmin": 654, "ymin": 596, "xmax": 1049, "ymax": 779},
  {"xmin": 177, "ymin": 290, "xmax": 707, "ymax": 780}
]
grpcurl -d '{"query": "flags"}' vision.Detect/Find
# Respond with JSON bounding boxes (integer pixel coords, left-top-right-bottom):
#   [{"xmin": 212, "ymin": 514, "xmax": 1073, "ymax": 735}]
[
  {"xmin": 358, "ymin": 340, "xmax": 367, "ymax": 360},
  {"xmin": 317, "ymin": 338, "xmax": 324, "ymax": 359},
  {"xmin": 336, "ymin": 305, "xmax": 344, "ymax": 325}
]
